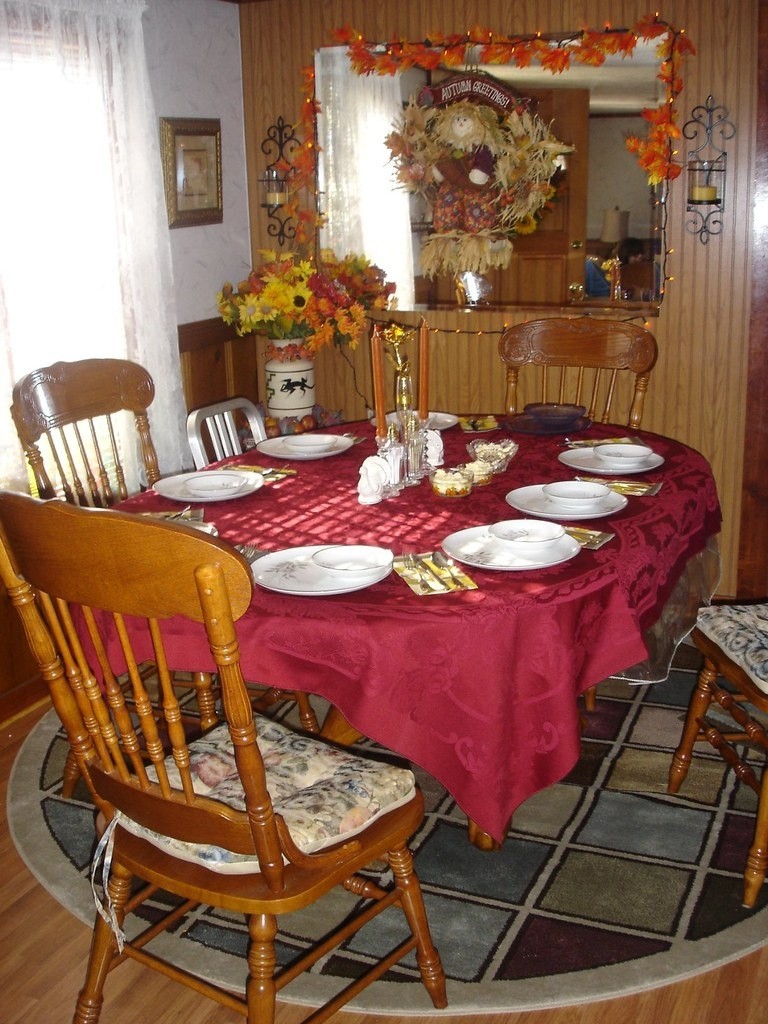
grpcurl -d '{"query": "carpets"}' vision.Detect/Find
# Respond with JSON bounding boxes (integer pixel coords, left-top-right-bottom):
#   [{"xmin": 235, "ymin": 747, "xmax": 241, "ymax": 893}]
[{"xmin": 8, "ymin": 638, "xmax": 768, "ymax": 1017}]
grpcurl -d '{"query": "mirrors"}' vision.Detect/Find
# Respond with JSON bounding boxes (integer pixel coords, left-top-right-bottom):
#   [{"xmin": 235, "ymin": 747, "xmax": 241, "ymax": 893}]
[{"xmin": 314, "ymin": 28, "xmax": 671, "ymax": 314}]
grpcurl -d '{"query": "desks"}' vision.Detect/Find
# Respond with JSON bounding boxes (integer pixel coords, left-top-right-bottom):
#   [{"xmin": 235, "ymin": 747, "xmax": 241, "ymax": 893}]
[{"xmin": 77, "ymin": 414, "xmax": 723, "ymax": 848}]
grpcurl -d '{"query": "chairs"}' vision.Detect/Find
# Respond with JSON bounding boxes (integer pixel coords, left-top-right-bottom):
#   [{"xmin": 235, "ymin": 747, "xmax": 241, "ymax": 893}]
[{"xmin": 0, "ymin": 317, "xmax": 768, "ymax": 1024}]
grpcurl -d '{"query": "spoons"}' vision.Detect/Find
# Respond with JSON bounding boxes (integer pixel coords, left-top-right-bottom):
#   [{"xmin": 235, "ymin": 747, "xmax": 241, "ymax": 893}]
[{"xmin": 431, "ymin": 550, "xmax": 464, "ymax": 589}]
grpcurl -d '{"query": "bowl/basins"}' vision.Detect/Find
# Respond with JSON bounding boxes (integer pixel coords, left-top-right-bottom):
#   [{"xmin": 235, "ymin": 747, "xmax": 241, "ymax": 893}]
[
  {"xmin": 182, "ymin": 474, "xmax": 248, "ymax": 498},
  {"xmin": 488, "ymin": 519, "xmax": 566, "ymax": 554},
  {"xmin": 310, "ymin": 545, "xmax": 394, "ymax": 578},
  {"xmin": 283, "ymin": 434, "xmax": 338, "ymax": 453},
  {"xmin": 542, "ymin": 481, "xmax": 611, "ymax": 509},
  {"xmin": 389, "ymin": 412, "xmax": 437, "ymax": 428},
  {"xmin": 593, "ymin": 443, "xmax": 653, "ymax": 466},
  {"xmin": 523, "ymin": 402, "xmax": 587, "ymax": 426}
]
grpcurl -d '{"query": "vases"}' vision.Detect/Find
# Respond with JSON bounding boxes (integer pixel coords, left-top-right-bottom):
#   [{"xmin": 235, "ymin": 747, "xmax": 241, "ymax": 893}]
[{"xmin": 265, "ymin": 339, "xmax": 316, "ymax": 420}]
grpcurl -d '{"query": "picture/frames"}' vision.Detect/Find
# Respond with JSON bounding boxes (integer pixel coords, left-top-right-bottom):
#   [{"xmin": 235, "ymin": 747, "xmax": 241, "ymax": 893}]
[{"xmin": 159, "ymin": 116, "xmax": 224, "ymax": 228}]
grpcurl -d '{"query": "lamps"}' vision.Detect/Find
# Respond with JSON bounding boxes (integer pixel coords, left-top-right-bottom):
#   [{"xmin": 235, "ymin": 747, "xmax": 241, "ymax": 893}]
[{"xmin": 601, "ymin": 205, "xmax": 630, "ymax": 245}]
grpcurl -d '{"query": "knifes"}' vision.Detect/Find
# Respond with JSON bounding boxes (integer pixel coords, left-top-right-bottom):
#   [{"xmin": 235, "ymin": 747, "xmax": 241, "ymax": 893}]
[{"xmin": 410, "ymin": 553, "xmax": 450, "ymax": 590}]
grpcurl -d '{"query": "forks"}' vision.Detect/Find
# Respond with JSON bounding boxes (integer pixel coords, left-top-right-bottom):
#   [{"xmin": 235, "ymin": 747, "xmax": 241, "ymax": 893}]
[
  {"xmin": 403, "ymin": 551, "xmax": 434, "ymax": 592},
  {"xmin": 240, "ymin": 543, "xmax": 258, "ymax": 558}
]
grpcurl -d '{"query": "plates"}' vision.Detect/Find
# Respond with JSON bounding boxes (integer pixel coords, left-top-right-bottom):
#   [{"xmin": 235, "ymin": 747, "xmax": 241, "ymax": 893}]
[
  {"xmin": 175, "ymin": 521, "xmax": 219, "ymax": 539},
  {"xmin": 249, "ymin": 546, "xmax": 392, "ymax": 596},
  {"xmin": 370, "ymin": 410, "xmax": 459, "ymax": 430},
  {"xmin": 505, "ymin": 484, "xmax": 629, "ymax": 521},
  {"xmin": 441, "ymin": 525, "xmax": 581, "ymax": 571},
  {"xmin": 152, "ymin": 470, "xmax": 264, "ymax": 502},
  {"xmin": 256, "ymin": 434, "xmax": 354, "ymax": 460},
  {"xmin": 503, "ymin": 412, "xmax": 592, "ymax": 435},
  {"xmin": 558, "ymin": 448, "xmax": 665, "ymax": 475}
]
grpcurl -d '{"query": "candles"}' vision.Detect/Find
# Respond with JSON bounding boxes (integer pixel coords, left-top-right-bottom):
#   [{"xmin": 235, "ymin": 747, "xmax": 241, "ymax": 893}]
[
  {"xmin": 370, "ymin": 323, "xmax": 387, "ymax": 437},
  {"xmin": 693, "ymin": 185, "xmax": 718, "ymax": 201},
  {"xmin": 266, "ymin": 192, "xmax": 285, "ymax": 204},
  {"xmin": 419, "ymin": 316, "xmax": 430, "ymax": 420}
]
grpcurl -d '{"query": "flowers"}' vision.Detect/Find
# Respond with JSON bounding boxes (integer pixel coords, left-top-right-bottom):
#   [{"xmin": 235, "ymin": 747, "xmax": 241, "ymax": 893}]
[
  {"xmin": 215, "ymin": 251, "xmax": 369, "ymax": 361},
  {"xmin": 515, "ymin": 166, "xmax": 566, "ymax": 236},
  {"xmin": 319, "ymin": 247, "xmax": 400, "ymax": 313},
  {"xmin": 237, "ymin": 401, "xmax": 345, "ymax": 442}
]
